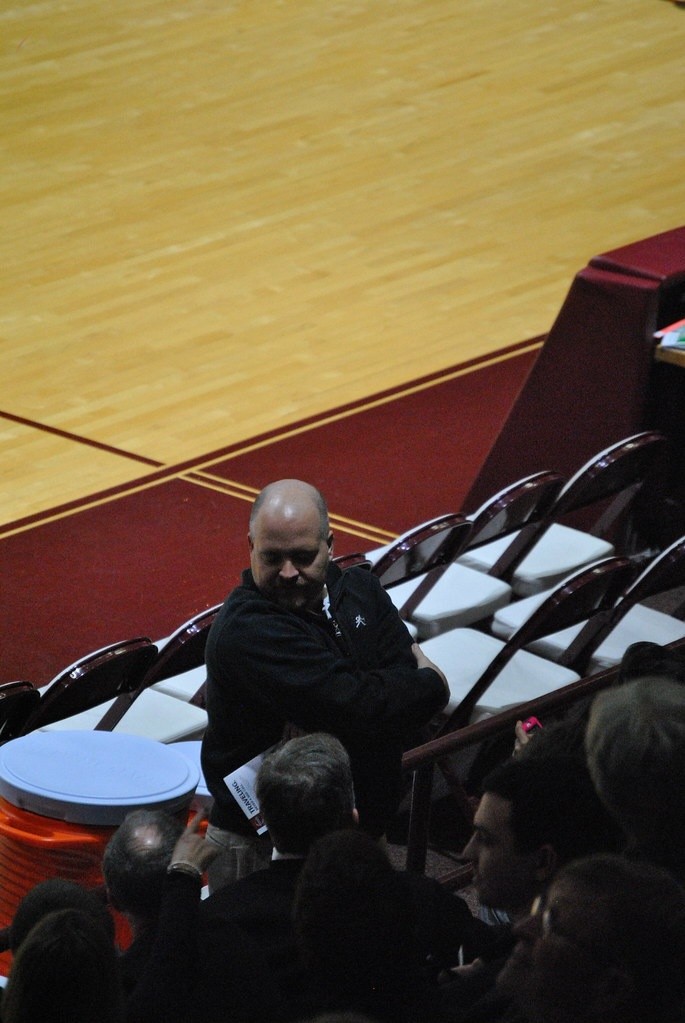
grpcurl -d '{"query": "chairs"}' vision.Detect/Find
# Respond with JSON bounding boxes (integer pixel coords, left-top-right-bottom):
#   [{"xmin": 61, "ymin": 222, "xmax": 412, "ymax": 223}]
[{"xmin": 0, "ymin": 430, "xmax": 685, "ymax": 830}]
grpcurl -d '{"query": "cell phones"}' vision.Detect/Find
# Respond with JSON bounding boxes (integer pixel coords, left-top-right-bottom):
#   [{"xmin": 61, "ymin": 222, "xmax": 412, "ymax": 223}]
[{"xmin": 522, "ymin": 718, "xmax": 543, "ymax": 736}]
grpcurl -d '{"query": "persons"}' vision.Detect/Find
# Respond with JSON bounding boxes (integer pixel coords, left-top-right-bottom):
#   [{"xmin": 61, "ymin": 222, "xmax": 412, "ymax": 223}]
[
  {"xmin": 200, "ymin": 478, "xmax": 450, "ymax": 896},
  {"xmin": 0, "ymin": 641, "xmax": 685, "ymax": 1023}
]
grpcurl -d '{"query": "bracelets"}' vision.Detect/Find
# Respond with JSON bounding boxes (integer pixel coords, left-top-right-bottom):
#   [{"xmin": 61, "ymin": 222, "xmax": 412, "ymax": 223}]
[{"xmin": 167, "ymin": 862, "xmax": 204, "ymax": 876}]
